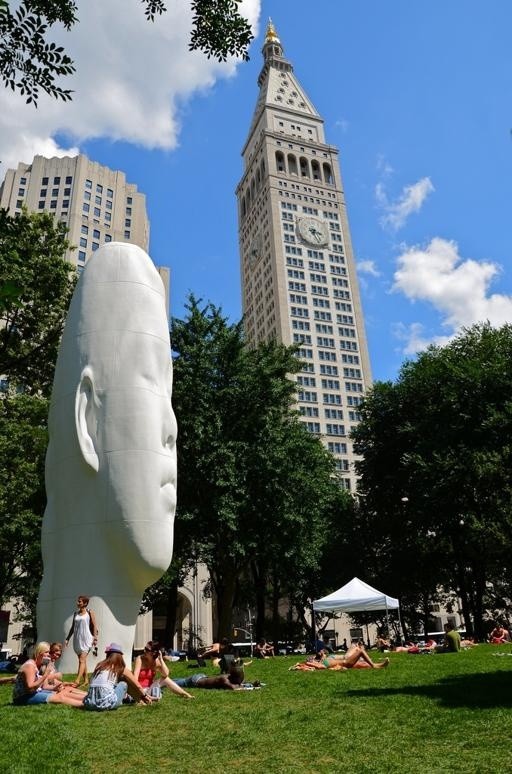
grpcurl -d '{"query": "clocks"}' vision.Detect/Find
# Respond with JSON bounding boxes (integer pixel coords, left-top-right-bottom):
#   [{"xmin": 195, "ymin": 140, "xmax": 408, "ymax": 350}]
[{"xmin": 294, "ymin": 212, "xmax": 329, "ymax": 249}]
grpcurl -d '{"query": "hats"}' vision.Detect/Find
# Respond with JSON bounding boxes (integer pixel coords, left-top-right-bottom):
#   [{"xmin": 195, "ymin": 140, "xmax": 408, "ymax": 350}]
[{"xmin": 105, "ymin": 643, "xmax": 124, "ymax": 654}]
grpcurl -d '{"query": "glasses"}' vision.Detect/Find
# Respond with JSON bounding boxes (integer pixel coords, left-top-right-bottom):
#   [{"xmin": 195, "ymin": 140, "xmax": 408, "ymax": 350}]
[{"xmin": 145, "ymin": 646, "xmax": 155, "ymax": 653}]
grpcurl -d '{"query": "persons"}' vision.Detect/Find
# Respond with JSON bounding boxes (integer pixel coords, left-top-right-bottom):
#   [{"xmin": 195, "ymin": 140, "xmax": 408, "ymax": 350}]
[
  {"xmin": 257, "ymin": 638, "xmax": 274, "ymax": 658},
  {"xmin": 318, "ymin": 634, "xmax": 436, "ymax": 653},
  {"xmin": 171, "ymin": 668, "xmax": 264, "ymax": 690},
  {"xmin": 33, "ymin": 242, "xmax": 181, "ymax": 673},
  {"xmin": 461, "ymin": 640, "xmax": 474, "ymax": 646},
  {"xmin": 1, "ymin": 656, "xmax": 18, "ymax": 673},
  {"xmin": 307, "ymin": 650, "xmax": 389, "ymax": 669},
  {"xmin": 64, "ymin": 595, "xmax": 99, "ymax": 686},
  {"xmin": 12, "ymin": 641, "xmax": 194, "ymax": 710},
  {"xmin": 487, "ymin": 624, "xmax": 509, "ymax": 643},
  {"xmin": 436, "ymin": 623, "xmax": 460, "ymax": 653},
  {"xmin": 188, "ymin": 637, "xmax": 228, "ymax": 668}
]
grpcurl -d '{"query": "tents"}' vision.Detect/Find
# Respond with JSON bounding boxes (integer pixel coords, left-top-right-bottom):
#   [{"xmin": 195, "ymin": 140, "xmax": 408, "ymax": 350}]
[{"xmin": 313, "ymin": 577, "xmax": 403, "ymax": 645}]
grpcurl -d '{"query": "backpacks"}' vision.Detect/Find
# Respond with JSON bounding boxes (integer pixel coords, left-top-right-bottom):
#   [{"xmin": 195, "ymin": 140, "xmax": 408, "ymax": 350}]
[{"xmin": 74, "ymin": 609, "xmax": 99, "ymax": 636}]
